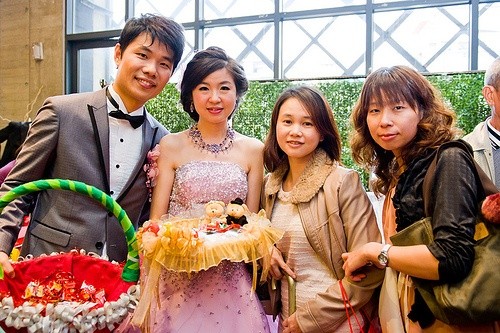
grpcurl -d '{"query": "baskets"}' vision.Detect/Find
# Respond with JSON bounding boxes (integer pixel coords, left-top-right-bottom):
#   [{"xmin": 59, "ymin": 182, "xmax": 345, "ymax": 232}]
[{"xmin": 0, "ymin": 178, "xmax": 140, "ymax": 333}]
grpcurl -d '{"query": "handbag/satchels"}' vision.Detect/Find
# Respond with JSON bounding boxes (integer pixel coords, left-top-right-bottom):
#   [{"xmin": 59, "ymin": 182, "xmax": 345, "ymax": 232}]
[
  {"xmin": 338, "ymin": 278, "xmax": 381, "ymax": 333},
  {"xmin": 390, "ymin": 140, "xmax": 500, "ymax": 327}
]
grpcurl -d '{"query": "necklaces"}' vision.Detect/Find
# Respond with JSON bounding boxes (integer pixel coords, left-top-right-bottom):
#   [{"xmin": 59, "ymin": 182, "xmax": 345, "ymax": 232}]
[{"xmin": 189, "ymin": 122, "xmax": 236, "ymax": 157}]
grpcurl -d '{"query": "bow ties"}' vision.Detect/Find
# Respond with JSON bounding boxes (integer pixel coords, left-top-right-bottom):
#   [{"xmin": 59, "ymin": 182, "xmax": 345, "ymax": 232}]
[{"xmin": 109, "ymin": 109, "xmax": 146, "ymax": 129}]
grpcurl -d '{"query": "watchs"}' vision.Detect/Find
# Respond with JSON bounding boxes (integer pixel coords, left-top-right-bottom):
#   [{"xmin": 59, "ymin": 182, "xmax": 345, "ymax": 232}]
[{"xmin": 377, "ymin": 244, "xmax": 392, "ymax": 269}]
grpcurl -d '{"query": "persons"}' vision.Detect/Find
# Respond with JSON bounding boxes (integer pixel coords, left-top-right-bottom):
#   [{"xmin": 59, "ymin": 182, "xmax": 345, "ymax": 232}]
[
  {"xmin": 258, "ymin": 86, "xmax": 388, "ymax": 333},
  {"xmin": 458, "ymin": 56, "xmax": 500, "ymax": 191},
  {"xmin": 0, "ymin": 121, "xmax": 32, "ymax": 167},
  {"xmin": 135, "ymin": 46, "xmax": 267, "ymax": 333},
  {"xmin": 0, "ymin": 12, "xmax": 184, "ymax": 282},
  {"xmin": 341, "ymin": 65, "xmax": 500, "ymax": 333}
]
